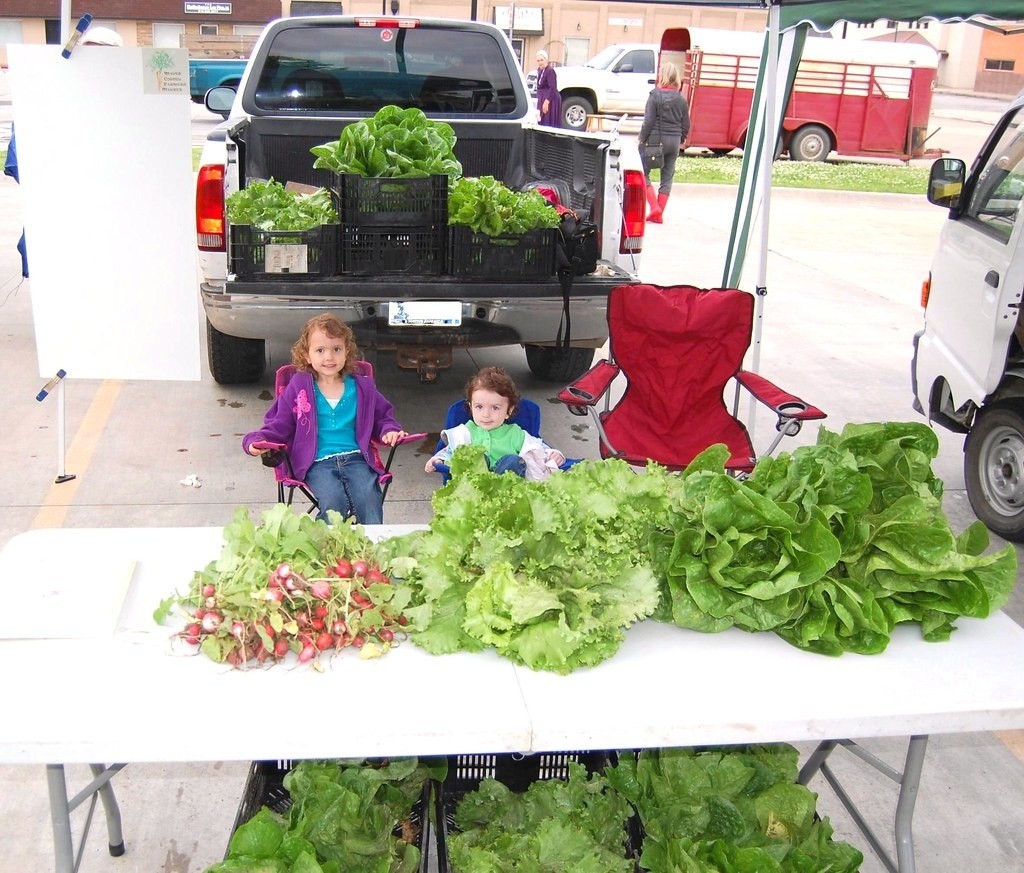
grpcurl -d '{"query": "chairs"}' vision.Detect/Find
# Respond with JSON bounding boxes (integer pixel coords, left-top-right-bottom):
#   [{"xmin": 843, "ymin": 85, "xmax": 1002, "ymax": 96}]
[
  {"xmin": 252, "ymin": 359, "xmax": 429, "ymax": 515},
  {"xmin": 556, "ymin": 284, "xmax": 827, "ymax": 481},
  {"xmin": 433, "ymin": 398, "xmax": 586, "ymax": 487}
]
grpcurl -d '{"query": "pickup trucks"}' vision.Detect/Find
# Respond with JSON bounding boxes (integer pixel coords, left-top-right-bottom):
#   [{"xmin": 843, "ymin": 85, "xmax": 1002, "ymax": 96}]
[
  {"xmin": 196, "ymin": 16, "xmax": 649, "ymax": 387},
  {"xmin": 190, "ymin": 48, "xmax": 482, "ymax": 120}
]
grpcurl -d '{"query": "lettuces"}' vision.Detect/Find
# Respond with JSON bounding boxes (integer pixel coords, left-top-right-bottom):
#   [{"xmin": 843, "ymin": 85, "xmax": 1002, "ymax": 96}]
[
  {"xmin": 392, "ymin": 444, "xmax": 685, "ymax": 675},
  {"xmin": 447, "ymin": 759, "xmax": 636, "ymax": 873},
  {"xmin": 222, "ymin": 175, "xmax": 338, "ymax": 261}
]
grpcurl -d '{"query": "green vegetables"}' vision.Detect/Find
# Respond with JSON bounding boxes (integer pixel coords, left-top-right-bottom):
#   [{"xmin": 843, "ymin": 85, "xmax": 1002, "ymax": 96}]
[
  {"xmin": 444, "ymin": 173, "xmax": 561, "ymax": 246},
  {"xmin": 642, "ymin": 421, "xmax": 1018, "ymax": 659},
  {"xmin": 603, "ymin": 743, "xmax": 866, "ymax": 873},
  {"xmin": 309, "ymin": 104, "xmax": 463, "ymax": 213},
  {"xmin": 201, "ymin": 756, "xmax": 449, "ymax": 873}
]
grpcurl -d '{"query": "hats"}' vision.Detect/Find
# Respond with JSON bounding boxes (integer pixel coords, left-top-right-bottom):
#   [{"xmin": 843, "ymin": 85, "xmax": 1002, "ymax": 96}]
[{"xmin": 81, "ymin": 27, "xmax": 123, "ymax": 47}]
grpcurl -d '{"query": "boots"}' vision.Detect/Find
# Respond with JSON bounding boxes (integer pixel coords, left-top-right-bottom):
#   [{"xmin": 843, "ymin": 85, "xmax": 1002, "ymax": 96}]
[
  {"xmin": 650, "ymin": 194, "xmax": 668, "ymax": 223},
  {"xmin": 645, "ymin": 185, "xmax": 662, "ymax": 221}
]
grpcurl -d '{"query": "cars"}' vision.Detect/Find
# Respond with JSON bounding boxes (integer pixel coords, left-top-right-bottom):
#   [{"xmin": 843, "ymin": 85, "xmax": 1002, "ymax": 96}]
[{"xmin": 525, "ymin": 43, "xmax": 662, "ymax": 131}]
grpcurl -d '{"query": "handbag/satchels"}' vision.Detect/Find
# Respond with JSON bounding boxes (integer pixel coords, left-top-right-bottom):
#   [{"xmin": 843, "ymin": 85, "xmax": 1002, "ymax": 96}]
[{"xmin": 644, "ymin": 146, "xmax": 664, "ymax": 169}]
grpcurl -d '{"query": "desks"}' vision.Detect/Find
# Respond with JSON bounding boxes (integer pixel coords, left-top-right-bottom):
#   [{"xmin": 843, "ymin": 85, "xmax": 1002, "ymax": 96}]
[{"xmin": 2, "ymin": 524, "xmax": 1024, "ymax": 873}]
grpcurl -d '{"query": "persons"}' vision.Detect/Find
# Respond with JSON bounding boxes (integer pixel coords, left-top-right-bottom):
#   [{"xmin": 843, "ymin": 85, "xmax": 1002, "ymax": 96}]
[
  {"xmin": 536, "ymin": 50, "xmax": 562, "ymax": 129},
  {"xmin": 425, "ymin": 367, "xmax": 565, "ymax": 483},
  {"xmin": 3, "ymin": 28, "xmax": 123, "ymax": 277},
  {"xmin": 239, "ymin": 311, "xmax": 410, "ymax": 525},
  {"xmin": 637, "ymin": 62, "xmax": 690, "ymax": 223}
]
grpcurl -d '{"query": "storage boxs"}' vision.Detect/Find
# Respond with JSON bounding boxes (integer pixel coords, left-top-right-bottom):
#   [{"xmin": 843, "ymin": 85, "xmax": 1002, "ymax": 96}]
[
  {"xmin": 227, "ymin": 170, "xmax": 559, "ymax": 282},
  {"xmin": 222, "ymin": 745, "xmax": 832, "ymax": 873}
]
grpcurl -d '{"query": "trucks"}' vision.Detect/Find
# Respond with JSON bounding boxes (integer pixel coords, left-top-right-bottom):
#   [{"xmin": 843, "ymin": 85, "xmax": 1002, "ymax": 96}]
[{"xmin": 910, "ymin": 96, "xmax": 1024, "ymax": 541}]
